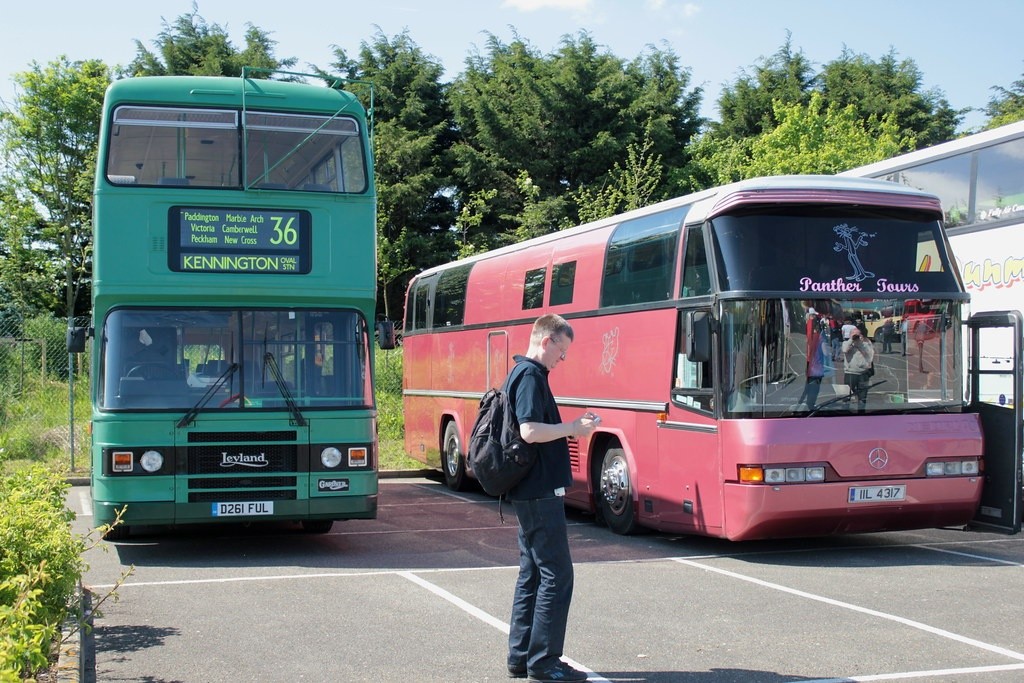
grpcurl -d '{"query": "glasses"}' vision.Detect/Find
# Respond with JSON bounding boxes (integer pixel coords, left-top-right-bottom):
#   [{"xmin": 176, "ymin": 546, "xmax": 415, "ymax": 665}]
[{"xmin": 549, "ymin": 337, "xmax": 567, "ymax": 359}]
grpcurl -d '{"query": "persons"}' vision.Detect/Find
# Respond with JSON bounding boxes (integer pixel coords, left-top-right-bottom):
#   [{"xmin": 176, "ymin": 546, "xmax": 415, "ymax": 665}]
[
  {"xmin": 801, "ymin": 299, "xmax": 875, "ymax": 412},
  {"xmin": 914, "ymin": 317, "xmax": 927, "ymax": 373},
  {"xmin": 880, "ymin": 315, "xmax": 912, "ymax": 356},
  {"xmin": 735, "ymin": 325, "xmax": 756, "ymax": 406},
  {"xmin": 503, "ymin": 314, "xmax": 602, "ymax": 683}
]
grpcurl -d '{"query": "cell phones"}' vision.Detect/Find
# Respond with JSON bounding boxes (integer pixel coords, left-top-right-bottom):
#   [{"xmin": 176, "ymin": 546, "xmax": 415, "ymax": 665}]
[{"xmin": 593, "ymin": 417, "xmax": 601, "ymax": 424}]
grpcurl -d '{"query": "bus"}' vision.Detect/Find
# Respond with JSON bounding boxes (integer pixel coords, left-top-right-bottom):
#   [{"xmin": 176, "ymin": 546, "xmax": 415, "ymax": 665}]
[
  {"xmin": 66, "ymin": 65, "xmax": 396, "ymax": 541},
  {"xmin": 837, "ymin": 118, "xmax": 1023, "ymax": 410},
  {"xmin": 402, "ymin": 174, "xmax": 988, "ymax": 542}
]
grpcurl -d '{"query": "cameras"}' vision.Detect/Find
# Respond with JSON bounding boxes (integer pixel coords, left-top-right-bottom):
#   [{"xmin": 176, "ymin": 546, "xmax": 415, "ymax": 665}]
[{"xmin": 852, "ymin": 334, "xmax": 860, "ymax": 341}]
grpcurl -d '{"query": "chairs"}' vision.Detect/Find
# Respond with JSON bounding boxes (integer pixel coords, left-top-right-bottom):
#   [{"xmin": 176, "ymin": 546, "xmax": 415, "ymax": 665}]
[
  {"xmin": 159, "ymin": 176, "xmax": 189, "ymax": 184},
  {"xmin": 257, "ymin": 183, "xmax": 288, "ymax": 188},
  {"xmin": 304, "ymin": 183, "xmax": 330, "ymax": 191},
  {"xmin": 197, "ymin": 359, "xmax": 294, "ymax": 391},
  {"xmin": 124, "ymin": 345, "xmax": 175, "ymax": 377},
  {"xmin": 107, "ymin": 174, "xmax": 137, "ymax": 184}
]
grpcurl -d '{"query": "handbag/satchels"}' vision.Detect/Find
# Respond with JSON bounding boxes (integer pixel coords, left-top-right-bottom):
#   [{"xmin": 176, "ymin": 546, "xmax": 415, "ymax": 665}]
[{"xmin": 861, "ymin": 367, "xmax": 875, "ymax": 382}]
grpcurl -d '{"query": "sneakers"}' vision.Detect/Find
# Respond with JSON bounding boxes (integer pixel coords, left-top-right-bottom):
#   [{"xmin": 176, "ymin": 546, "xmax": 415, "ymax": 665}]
[
  {"xmin": 506, "ymin": 662, "xmax": 528, "ymax": 678},
  {"xmin": 527, "ymin": 660, "xmax": 588, "ymax": 683}
]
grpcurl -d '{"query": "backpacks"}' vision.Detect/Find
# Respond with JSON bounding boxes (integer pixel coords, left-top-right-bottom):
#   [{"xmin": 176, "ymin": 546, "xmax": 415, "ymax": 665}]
[{"xmin": 467, "ymin": 361, "xmax": 540, "ymax": 526}]
